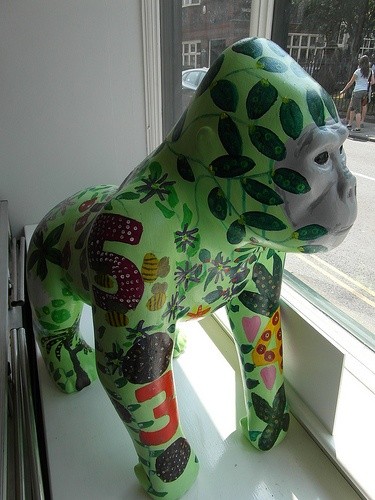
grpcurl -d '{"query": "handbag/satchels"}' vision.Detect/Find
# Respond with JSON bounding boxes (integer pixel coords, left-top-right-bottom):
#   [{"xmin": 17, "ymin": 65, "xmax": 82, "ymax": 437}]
[{"xmin": 363, "ymin": 95, "xmax": 368, "ymax": 105}]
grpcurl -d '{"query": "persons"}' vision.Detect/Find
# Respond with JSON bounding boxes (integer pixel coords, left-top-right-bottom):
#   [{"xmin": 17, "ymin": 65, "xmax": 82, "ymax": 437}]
[
  {"xmin": 348, "ymin": 61, "xmax": 374, "ymax": 131},
  {"xmin": 340, "ymin": 54, "xmax": 375, "ymax": 133}
]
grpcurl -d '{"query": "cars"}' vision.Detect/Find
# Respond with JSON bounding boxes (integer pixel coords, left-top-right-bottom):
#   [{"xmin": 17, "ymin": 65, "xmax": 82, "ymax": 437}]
[{"xmin": 181, "ymin": 68, "xmax": 209, "ymax": 115}]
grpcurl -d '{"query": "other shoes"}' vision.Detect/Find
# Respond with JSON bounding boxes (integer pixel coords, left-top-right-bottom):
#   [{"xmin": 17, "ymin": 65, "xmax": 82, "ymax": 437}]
[
  {"xmin": 346, "ymin": 124, "xmax": 352, "ymax": 129},
  {"xmin": 353, "ymin": 127, "xmax": 361, "ymax": 132}
]
